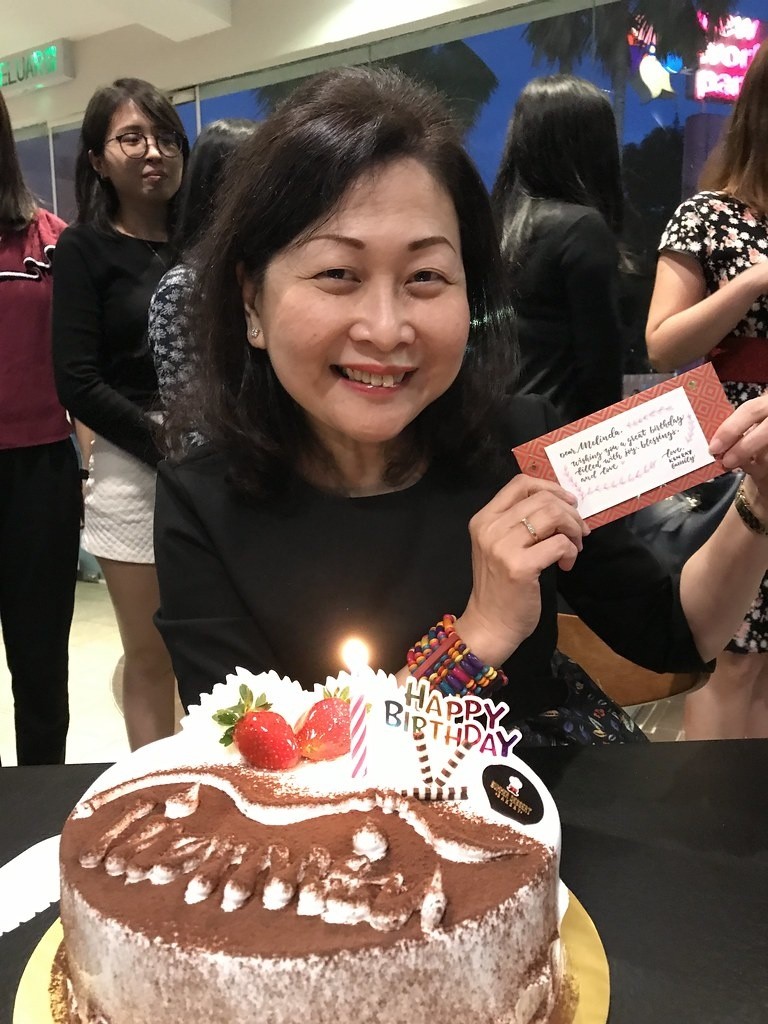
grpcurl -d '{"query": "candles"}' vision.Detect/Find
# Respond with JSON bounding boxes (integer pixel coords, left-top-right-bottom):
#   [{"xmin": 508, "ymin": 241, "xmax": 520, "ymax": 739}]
[{"xmin": 342, "ymin": 635, "xmax": 375, "ymax": 777}]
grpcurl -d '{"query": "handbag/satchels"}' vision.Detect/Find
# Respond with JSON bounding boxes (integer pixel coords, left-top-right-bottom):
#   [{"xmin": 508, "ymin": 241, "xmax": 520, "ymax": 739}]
[{"xmin": 622, "ymin": 471, "xmax": 746, "ymax": 574}]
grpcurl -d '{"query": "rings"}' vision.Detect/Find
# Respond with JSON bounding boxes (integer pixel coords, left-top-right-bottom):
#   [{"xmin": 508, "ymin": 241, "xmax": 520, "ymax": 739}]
[{"xmin": 522, "ymin": 518, "xmax": 540, "ymax": 543}]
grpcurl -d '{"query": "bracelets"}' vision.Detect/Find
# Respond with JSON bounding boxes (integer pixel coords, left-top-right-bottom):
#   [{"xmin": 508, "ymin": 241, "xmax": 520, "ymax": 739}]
[
  {"xmin": 407, "ymin": 612, "xmax": 509, "ymax": 699},
  {"xmin": 79, "ymin": 467, "xmax": 90, "ymax": 480},
  {"xmin": 734, "ymin": 479, "xmax": 768, "ymax": 537}
]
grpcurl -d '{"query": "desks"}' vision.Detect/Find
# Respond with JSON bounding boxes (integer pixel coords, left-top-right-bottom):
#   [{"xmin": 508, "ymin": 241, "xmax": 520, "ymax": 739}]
[{"xmin": 0, "ymin": 735, "xmax": 768, "ymax": 1024}]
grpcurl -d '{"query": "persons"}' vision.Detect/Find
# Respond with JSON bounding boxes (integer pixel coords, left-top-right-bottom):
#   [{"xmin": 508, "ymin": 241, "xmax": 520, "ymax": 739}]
[
  {"xmin": 154, "ymin": 64, "xmax": 768, "ymax": 745},
  {"xmin": 636, "ymin": 40, "xmax": 768, "ymax": 743},
  {"xmin": 148, "ymin": 117, "xmax": 264, "ymax": 465},
  {"xmin": 0, "ymin": 89, "xmax": 82, "ymax": 769},
  {"xmin": 488, "ymin": 74, "xmax": 644, "ymax": 482},
  {"xmin": 54, "ymin": 75, "xmax": 192, "ymax": 749}
]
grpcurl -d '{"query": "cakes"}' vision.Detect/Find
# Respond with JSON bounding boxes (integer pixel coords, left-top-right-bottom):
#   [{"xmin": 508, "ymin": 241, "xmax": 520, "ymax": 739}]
[{"xmin": 58, "ymin": 684, "xmax": 562, "ymax": 1024}]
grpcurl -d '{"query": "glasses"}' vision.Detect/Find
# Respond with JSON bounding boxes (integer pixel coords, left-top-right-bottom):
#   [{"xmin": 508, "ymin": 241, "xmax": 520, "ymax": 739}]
[{"xmin": 106, "ymin": 131, "xmax": 182, "ymax": 159}]
